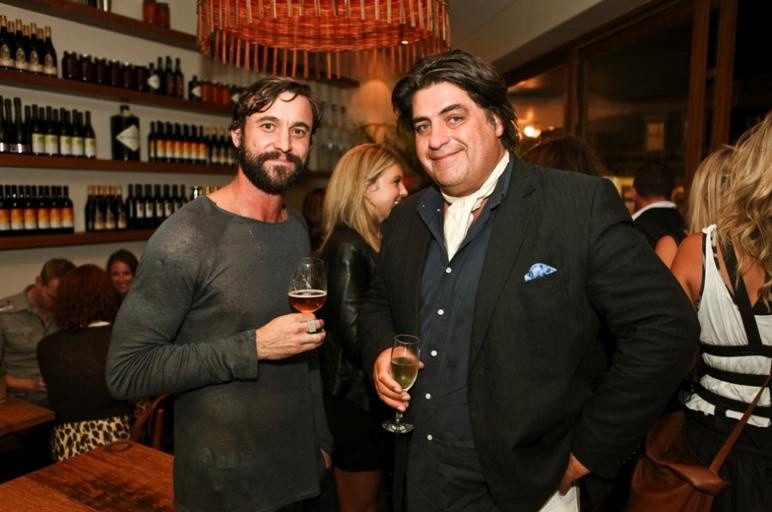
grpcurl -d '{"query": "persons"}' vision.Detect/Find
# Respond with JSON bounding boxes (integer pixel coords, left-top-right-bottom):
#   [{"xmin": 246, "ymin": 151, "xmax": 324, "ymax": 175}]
[
  {"xmin": 670, "ymin": 112, "xmax": 771, "ymax": 512},
  {"xmin": 106, "ymin": 248, "xmax": 139, "ymax": 311},
  {"xmin": 359, "ymin": 48, "xmax": 701, "ymax": 512},
  {"xmin": 654, "ymin": 144, "xmax": 735, "ymax": 271},
  {"xmin": 297, "ymin": 187, "xmax": 326, "ymax": 249},
  {"xmin": 3, "ymin": 257, "xmax": 78, "ymax": 409},
  {"xmin": 626, "ymin": 159, "xmax": 685, "ymax": 244},
  {"xmin": 106, "ymin": 77, "xmax": 341, "ymax": 511},
  {"xmin": 519, "ymin": 134, "xmax": 606, "ymax": 178},
  {"xmin": 315, "ymin": 144, "xmax": 410, "ymax": 472},
  {"xmin": 37, "ymin": 263, "xmax": 151, "ymax": 464}
]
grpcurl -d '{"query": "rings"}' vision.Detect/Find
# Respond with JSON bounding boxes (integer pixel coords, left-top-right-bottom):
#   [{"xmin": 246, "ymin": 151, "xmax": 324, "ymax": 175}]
[{"xmin": 305, "ymin": 319, "xmax": 315, "ymax": 336}]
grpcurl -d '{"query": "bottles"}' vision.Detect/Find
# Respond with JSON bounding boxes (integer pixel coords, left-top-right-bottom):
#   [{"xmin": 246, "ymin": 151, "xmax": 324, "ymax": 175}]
[
  {"xmin": 85, "ymin": 185, "xmax": 95, "ymax": 230},
  {"xmin": 24, "ymin": 24, "xmax": 29, "ymax": 49},
  {"xmin": 0, "ymin": 184, "xmax": 10, "ymax": 235},
  {"xmin": 146, "ymin": 63, "xmax": 160, "ymax": 92},
  {"xmin": 13, "ymin": 98, "xmax": 27, "ymax": 154},
  {"xmin": 227, "ymin": 130, "xmax": 237, "ymax": 166},
  {"xmin": 174, "ymin": 122, "xmax": 182, "ymax": 162},
  {"xmin": 153, "ymin": 184, "xmax": 163, "ymax": 227},
  {"xmin": 155, "ymin": 121, "xmax": 166, "ymax": 161},
  {"xmin": 210, "ymin": 128, "xmax": 218, "ymax": 164},
  {"xmin": 218, "ymin": 128, "xmax": 227, "ymax": 165},
  {"xmin": 5, "ymin": 99, "xmax": 14, "ymax": 153},
  {"xmin": 84, "ymin": 111, "xmax": 96, "ymax": 158},
  {"xmin": 0, "ymin": 14, "xmax": 13, "ymax": 70},
  {"xmin": 66, "ymin": 110, "xmax": 72, "ymax": 124},
  {"xmin": 121, "ymin": 61, "xmax": 135, "ymax": 88},
  {"xmin": 94, "ymin": 57, "xmax": 109, "ymax": 83},
  {"xmin": 19, "ymin": 185, "xmax": 24, "ymax": 203},
  {"xmin": 14, "ymin": 19, "xmax": 28, "ymax": 71},
  {"xmin": 93, "ymin": 185, "xmax": 104, "ymax": 230},
  {"xmin": 179, "ymin": 185, "xmax": 188, "ymax": 207},
  {"xmin": 162, "ymin": 184, "xmax": 171, "ymax": 218},
  {"xmin": 29, "ymin": 22, "xmax": 43, "ymax": 74},
  {"xmin": 53, "ymin": 109, "xmax": 58, "ymax": 124},
  {"xmin": 205, "ymin": 128, "xmax": 210, "ymax": 144},
  {"xmin": 192, "ymin": 184, "xmax": 225, "ymax": 200},
  {"xmin": 189, "ymin": 75, "xmax": 201, "ymax": 99},
  {"xmin": 24, "ymin": 185, "xmax": 38, "ymax": 233},
  {"xmin": 40, "ymin": 107, "xmax": 45, "ymax": 122},
  {"xmin": 144, "ymin": 184, "xmax": 154, "ymax": 227},
  {"xmin": 221, "ymin": 82, "xmax": 231, "ymax": 103},
  {"xmin": 108, "ymin": 60, "xmax": 122, "ymax": 86},
  {"xmin": 190, "ymin": 125, "xmax": 199, "ymax": 164},
  {"xmin": 165, "ymin": 56, "xmax": 173, "ymax": 96},
  {"xmin": 10, "ymin": 185, "xmax": 24, "ymax": 234},
  {"xmin": 45, "ymin": 185, "xmax": 50, "ymax": 198},
  {"xmin": 116, "ymin": 186, "xmax": 126, "ymax": 229},
  {"xmin": 156, "ymin": 57, "xmax": 164, "ymax": 78},
  {"xmin": 38, "ymin": 185, "xmax": 50, "ymax": 233},
  {"xmin": 23, "ymin": 105, "xmax": 31, "ymax": 128},
  {"xmin": 148, "ymin": 121, "xmax": 155, "ymax": 160},
  {"xmin": 330, "ymin": 103, "xmax": 342, "ymax": 168},
  {"xmin": 53, "ymin": 45, "xmax": 58, "ymax": 77},
  {"xmin": 0, "ymin": 95, "xmax": 6, "ymax": 152},
  {"xmin": 125, "ymin": 184, "xmax": 133, "ymax": 228},
  {"xmin": 30, "ymin": 104, "xmax": 45, "ymax": 154},
  {"xmin": 318, "ymin": 103, "xmax": 331, "ymax": 172},
  {"xmin": 36, "ymin": 29, "xmax": 43, "ymax": 54},
  {"xmin": 212, "ymin": 80, "xmax": 221, "ymax": 103},
  {"xmin": 231, "ymin": 84, "xmax": 239, "ymax": 106},
  {"xmin": 111, "ymin": 105, "xmax": 140, "ymax": 160},
  {"xmin": 31, "ymin": 185, "xmax": 38, "ymax": 205},
  {"xmin": 78, "ymin": 112, "xmax": 84, "ymax": 125},
  {"xmin": 342, "ymin": 106, "xmax": 353, "ymax": 153},
  {"xmin": 71, "ymin": 109, "xmax": 84, "ymax": 157},
  {"xmin": 166, "ymin": 122, "xmax": 174, "ymax": 163},
  {"xmin": 6, "ymin": 184, "xmax": 10, "ymax": 202},
  {"xmin": 46, "ymin": 106, "xmax": 59, "ymax": 156},
  {"xmin": 201, "ymin": 78, "xmax": 212, "ymax": 103},
  {"xmin": 7, "ymin": 21, "xmax": 17, "ymax": 54},
  {"xmin": 79, "ymin": 54, "xmax": 94, "ymax": 80},
  {"xmin": 199, "ymin": 126, "xmax": 208, "ymax": 165},
  {"xmin": 50, "ymin": 186, "xmax": 61, "ymax": 233},
  {"xmin": 182, "ymin": 123, "xmax": 190, "ymax": 163},
  {"xmin": 59, "ymin": 108, "xmax": 71, "ymax": 156},
  {"xmin": 61, "ymin": 186, "xmax": 74, "ymax": 233},
  {"xmin": 171, "ymin": 184, "xmax": 179, "ymax": 211},
  {"xmin": 104, "ymin": 185, "xmax": 116, "ymax": 229},
  {"xmin": 43, "ymin": 27, "xmax": 57, "ymax": 78},
  {"xmin": 61, "ymin": 51, "xmax": 78, "ymax": 79},
  {"xmin": 134, "ymin": 184, "xmax": 144, "ymax": 228},
  {"xmin": 174, "ymin": 57, "xmax": 183, "ymax": 98},
  {"xmin": 57, "ymin": 186, "xmax": 61, "ymax": 197},
  {"xmin": 135, "ymin": 66, "xmax": 146, "ymax": 91}
]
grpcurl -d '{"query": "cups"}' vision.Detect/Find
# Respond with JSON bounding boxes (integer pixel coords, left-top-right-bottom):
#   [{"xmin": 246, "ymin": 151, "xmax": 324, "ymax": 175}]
[
  {"xmin": 285, "ymin": 259, "xmax": 329, "ymax": 313},
  {"xmin": 381, "ymin": 333, "xmax": 421, "ymax": 433}
]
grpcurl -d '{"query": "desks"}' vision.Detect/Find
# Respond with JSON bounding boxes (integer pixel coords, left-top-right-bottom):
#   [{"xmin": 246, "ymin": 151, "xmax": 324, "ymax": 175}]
[
  {"xmin": 0, "ymin": 394, "xmax": 55, "ymax": 482},
  {"xmin": 0, "ymin": 438, "xmax": 175, "ymax": 511}
]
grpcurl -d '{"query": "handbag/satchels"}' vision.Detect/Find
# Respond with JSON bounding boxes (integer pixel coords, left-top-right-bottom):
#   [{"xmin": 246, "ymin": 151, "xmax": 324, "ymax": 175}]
[{"xmin": 623, "ymin": 410, "xmax": 728, "ymax": 512}]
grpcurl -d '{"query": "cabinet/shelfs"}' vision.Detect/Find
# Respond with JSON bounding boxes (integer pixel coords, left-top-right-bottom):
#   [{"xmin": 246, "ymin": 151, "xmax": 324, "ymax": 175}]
[{"xmin": 0, "ymin": 0, "xmax": 359, "ymax": 248}]
